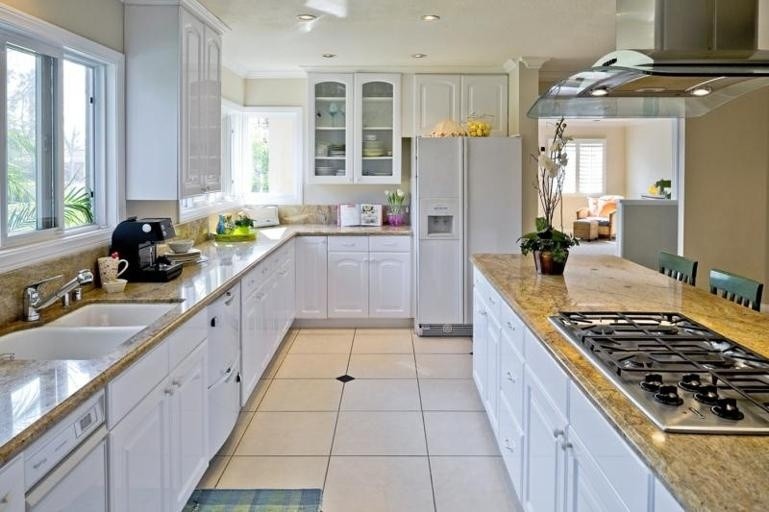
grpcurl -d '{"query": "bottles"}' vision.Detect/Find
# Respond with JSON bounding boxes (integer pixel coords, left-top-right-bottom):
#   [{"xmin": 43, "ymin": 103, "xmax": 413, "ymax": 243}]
[{"xmin": 217, "ymin": 214, "xmax": 234, "ymax": 234}]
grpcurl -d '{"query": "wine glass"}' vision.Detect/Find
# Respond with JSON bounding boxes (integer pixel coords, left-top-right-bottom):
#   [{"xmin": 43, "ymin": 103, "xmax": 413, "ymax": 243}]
[{"xmin": 315, "ymin": 101, "xmax": 345, "ymax": 127}]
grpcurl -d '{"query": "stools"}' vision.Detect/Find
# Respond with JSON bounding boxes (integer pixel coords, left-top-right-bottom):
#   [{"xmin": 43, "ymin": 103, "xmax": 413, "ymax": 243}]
[{"xmin": 573, "ymin": 220, "xmax": 599, "ymax": 242}]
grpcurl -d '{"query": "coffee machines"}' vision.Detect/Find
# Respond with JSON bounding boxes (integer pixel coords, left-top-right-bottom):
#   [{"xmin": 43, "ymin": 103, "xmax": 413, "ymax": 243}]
[{"xmin": 111, "ymin": 216, "xmax": 183, "ymax": 281}]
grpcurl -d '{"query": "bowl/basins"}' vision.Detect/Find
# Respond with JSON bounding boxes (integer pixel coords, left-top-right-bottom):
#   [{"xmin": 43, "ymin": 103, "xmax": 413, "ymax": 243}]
[
  {"xmin": 168, "ymin": 240, "xmax": 193, "ymax": 253},
  {"xmin": 103, "ymin": 279, "xmax": 126, "ymax": 293}
]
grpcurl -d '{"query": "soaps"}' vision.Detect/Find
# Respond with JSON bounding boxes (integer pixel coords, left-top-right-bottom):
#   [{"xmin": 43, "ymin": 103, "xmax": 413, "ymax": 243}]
[{"xmin": 101, "ymin": 278, "xmax": 127, "ymax": 293}]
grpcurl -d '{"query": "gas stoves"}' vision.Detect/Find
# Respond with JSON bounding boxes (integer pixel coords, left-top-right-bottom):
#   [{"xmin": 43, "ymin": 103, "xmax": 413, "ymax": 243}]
[{"xmin": 546, "ymin": 309, "xmax": 769, "ymax": 435}]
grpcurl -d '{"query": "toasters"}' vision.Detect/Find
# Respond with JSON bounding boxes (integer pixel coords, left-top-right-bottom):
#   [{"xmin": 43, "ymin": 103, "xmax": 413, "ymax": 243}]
[{"xmin": 242, "ymin": 207, "xmax": 279, "ymax": 227}]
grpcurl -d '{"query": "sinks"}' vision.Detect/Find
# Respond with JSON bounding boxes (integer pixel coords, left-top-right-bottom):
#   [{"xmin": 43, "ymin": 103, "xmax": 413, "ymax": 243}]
[
  {"xmin": 0, "ymin": 327, "xmax": 143, "ymax": 361},
  {"xmin": 49, "ymin": 300, "xmax": 184, "ymax": 327}
]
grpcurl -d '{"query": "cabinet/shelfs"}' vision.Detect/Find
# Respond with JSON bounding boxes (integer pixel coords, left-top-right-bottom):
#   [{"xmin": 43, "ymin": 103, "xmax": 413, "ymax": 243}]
[
  {"xmin": 495, "ymin": 299, "xmax": 524, "ymax": 504},
  {"xmin": 206, "ymin": 282, "xmax": 241, "ymax": 461},
  {"xmin": 305, "ymin": 72, "xmax": 402, "ymax": 184},
  {"xmin": 271, "ymin": 239, "xmax": 295, "ymax": 352},
  {"xmin": 328, "ymin": 236, "xmax": 412, "ymax": 320},
  {"xmin": 26, "ymin": 426, "xmax": 109, "ymax": 512},
  {"xmin": 472, "ymin": 265, "xmax": 501, "ymax": 418},
  {"xmin": 295, "ymin": 236, "xmax": 327, "ymax": 319},
  {"xmin": 522, "ymin": 330, "xmax": 686, "ymax": 512},
  {"xmin": 415, "ymin": 74, "xmax": 508, "ymax": 136},
  {"xmin": 241, "ymin": 259, "xmax": 271, "ymax": 401},
  {"xmin": 122, "ymin": 0, "xmax": 230, "ymax": 201},
  {"xmin": 105, "ymin": 308, "xmax": 208, "ymax": 512}
]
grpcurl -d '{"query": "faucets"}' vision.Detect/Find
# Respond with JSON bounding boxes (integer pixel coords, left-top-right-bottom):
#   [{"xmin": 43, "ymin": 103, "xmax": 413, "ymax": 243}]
[{"xmin": 25, "ymin": 267, "xmax": 94, "ymax": 322}]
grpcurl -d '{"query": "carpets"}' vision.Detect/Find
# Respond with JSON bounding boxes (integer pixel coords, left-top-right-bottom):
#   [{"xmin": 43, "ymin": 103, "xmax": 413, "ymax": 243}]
[{"xmin": 182, "ymin": 488, "xmax": 323, "ymax": 512}]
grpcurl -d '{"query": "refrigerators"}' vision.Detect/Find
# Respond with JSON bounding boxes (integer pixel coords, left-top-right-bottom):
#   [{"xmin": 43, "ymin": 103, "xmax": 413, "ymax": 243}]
[{"xmin": 410, "ymin": 138, "xmax": 523, "ymax": 338}]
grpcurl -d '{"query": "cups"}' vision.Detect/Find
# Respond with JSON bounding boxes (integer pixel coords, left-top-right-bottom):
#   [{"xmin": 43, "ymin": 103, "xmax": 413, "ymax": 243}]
[{"xmin": 98, "ymin": 256, "xmax": 128, "ymax": 286}]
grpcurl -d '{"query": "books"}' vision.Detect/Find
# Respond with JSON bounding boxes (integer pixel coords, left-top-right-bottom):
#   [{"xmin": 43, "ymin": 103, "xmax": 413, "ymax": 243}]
[{"xmin": 340, "ymin": 203, "xmax": 384, "ymax": 228}]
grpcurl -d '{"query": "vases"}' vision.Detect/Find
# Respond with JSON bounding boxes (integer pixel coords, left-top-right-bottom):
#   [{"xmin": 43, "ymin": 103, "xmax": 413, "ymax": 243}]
[
  {"xmin": 533, "ymin": 250, "xmax": 568, "ymax": 275},
  {"xmin": 387, "ymin": 213, "xmax": 406, "ymax": 226}
]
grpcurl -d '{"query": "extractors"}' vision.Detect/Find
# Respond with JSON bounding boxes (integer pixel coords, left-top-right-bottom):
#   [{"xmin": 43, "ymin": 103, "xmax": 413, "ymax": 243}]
[{"xmin": 526, "ymin": 0, "xmax": 769, "ymax": 120}]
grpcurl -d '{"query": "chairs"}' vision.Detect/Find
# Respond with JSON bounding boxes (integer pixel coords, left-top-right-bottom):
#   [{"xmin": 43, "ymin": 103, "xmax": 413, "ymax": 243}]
[
  {"xmin": 657, "ymin": 251, "xmax": 698, "ymax": 286},
  {"xmin": 709, "ymin": 267, "xmax": 763, "ymax": 312},
  {"xmin": 576, "ymin": 195, "xmax": 624, "ymax": 241}
]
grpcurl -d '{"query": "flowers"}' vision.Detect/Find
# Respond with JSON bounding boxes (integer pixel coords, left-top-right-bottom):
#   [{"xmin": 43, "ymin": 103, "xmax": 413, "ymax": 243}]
[
  {"xmin": 516, "ymin": 116, "xmax": 581, "ymax": 262},
  {"xmin": 384, "ymin": 189, "xmax": 407, "ymax": 213},
  {"xmin": 465, "ymin": 119, "xmax": 492, "ymax": 137}
]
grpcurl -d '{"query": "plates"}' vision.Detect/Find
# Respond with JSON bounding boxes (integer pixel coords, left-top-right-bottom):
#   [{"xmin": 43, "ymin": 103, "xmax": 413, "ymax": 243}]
[
  {"xmin": 315, "ymin": 140, "xmax": 392, "ymax": 175},
  {"xmin": 163, "ymin": 248, "xmax": 201, "ymax": 264}
]
